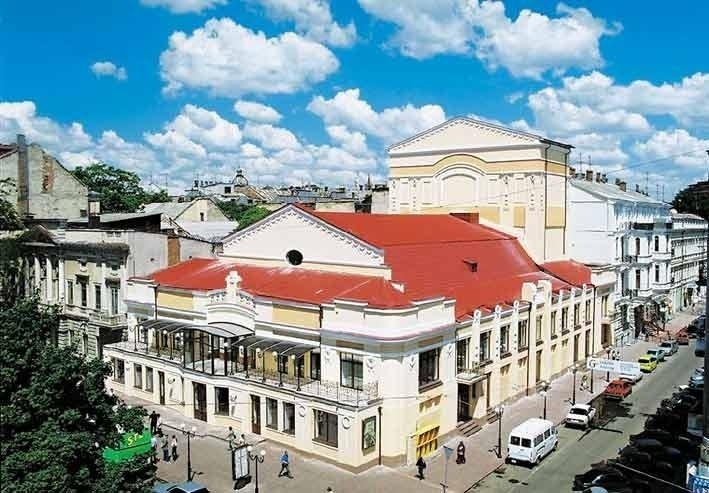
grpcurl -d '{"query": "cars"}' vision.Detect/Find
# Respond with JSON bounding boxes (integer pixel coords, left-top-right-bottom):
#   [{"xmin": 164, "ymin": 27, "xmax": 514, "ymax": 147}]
[
  {"xmin": 646, "ymin": 346, "xmax": 666, "ymax": 361},
  {"xmin": 600, "ymin": 377, "xmax": 633, "ymax": 401},
  {"xmin": 677, "ymin": 364, "xmax": 706, "ymax": 413},
  {"xmin": 676, "ymin": 312, "xmax": 706, "ymax": 356},
  {"xmin": 618, "ymin": 397, "xmax": 705, "ymax": 491},
  {"xmin": 619, "ymin": 370, "xmax": 644, "ymax": 384},
  {"xmin": 563, "ymin": 402, "xmax": 598, "ymax": 429},
  {"xmin": 142, "ymin": 478, "xmax": 211, "ymax": 492},
  {"xmin": 570, "ymin": 464, "xmax": 621, "ymax": 492},
  {"xmin": 637, "ymin": 353, "xmax": 658, "ymax": 372},
  {"xmin": 655, "ymin": 339, "xmax": 679, "ymax": 355}
]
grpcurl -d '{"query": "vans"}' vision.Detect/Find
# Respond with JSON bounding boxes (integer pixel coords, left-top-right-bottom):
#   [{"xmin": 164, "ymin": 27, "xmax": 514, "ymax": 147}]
[{"xmin": 506, "ymin": 416, "xmax": 561, "ymax": 467}]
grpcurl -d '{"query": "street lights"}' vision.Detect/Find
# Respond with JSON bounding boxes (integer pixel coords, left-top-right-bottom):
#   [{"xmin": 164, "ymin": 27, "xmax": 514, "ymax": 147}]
[
  {"xmin": 246, "ymin": 444, "xmax": 266, "ymax": 492},
  {"xmin": 494, "ymin": 402, "xmax": 506, "ymax": 458},
  {"xmin": 540, "ymin": 378, "xmax": 551, "ymax": 420},
  {"xmin": 571, "ymin": 343, "xmax": 613, "ymax": 406},
  {"xmin": 180, "ymin": 422, "xmax": 198, "ymax": 481}
]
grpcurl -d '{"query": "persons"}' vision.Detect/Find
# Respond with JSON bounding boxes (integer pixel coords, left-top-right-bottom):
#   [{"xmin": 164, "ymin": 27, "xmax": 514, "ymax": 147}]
[
  {"xmin": 416, "ymin": 456, "xmax": 426, "ymax": 480},
  {"xmin": 456, "ymin": 441, "xmax": 465, "ymax": 464},
  {"xmin": 326, "ymin": 487, "xmax": 333, "ymax": 493},
  {"xmin": 611, "ymin": 350, "xmax": 621, "ymax": 361},
  {"xmin": 109, "ymin": 388, "xmax": 177, "ymax": 464},
  {"xmin": 225, "ymin": 427, "xmax": 235, "ymax": 451},
  {"xmin": 278, "ymin": 451, "xmax": 294, "ymax": 479},
  {"xmin": 238, "ymin": 434, "xmax": 245, "ymax": 446}
]
